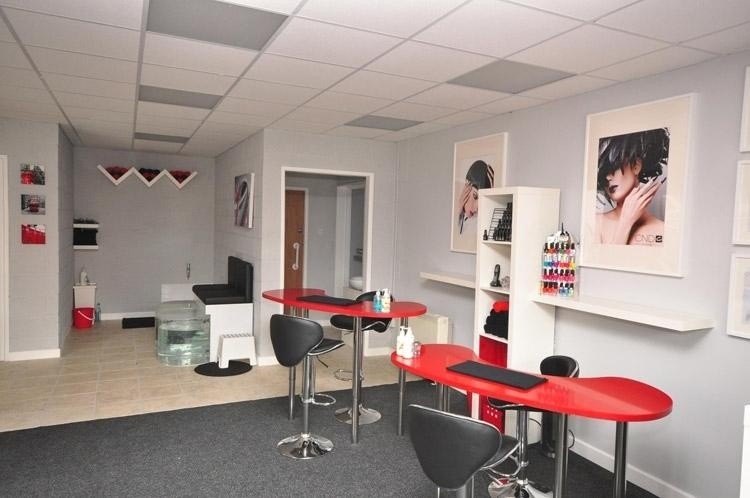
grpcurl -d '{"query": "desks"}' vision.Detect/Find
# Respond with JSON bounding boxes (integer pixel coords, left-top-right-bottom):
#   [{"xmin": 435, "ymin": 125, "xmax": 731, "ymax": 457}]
[
  {"xmin": 387, "ymin": 336, "xmax": 672, "ymax": 497},
  {"xmin": 258, "ymin": 286, "xmax": 428, "ymax": 446}
]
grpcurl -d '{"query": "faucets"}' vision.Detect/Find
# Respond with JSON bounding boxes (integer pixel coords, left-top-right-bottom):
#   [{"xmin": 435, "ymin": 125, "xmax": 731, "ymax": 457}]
[{"xmin": 185, "ymin": 264, "xmax": 190, "ymax": 281}]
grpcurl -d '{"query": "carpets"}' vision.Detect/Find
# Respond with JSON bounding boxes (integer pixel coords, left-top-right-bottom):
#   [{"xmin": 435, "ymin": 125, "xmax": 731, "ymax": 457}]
[
  {"xmin": 193, "ymin": 358, "xmax": 254, "ymax": 379},
  {"xmin": 0, "ymin": 375, "xmax": 663, "ymax": 497}
]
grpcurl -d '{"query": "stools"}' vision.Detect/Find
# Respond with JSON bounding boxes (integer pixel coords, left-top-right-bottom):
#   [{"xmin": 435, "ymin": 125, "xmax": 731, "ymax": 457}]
[{"xmin": 216, "ymin": 333, "xmax": 261, "ymax": 368}]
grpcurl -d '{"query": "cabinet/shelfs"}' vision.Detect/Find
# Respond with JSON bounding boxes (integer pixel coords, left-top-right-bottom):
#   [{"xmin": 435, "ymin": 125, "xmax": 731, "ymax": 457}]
[
  {"xmin": 472, "ymin": 186, "xmax": 563, "ymax": 448},
  {"xmin": 340, "ymin": 287, "xmax": 363, "ymax": 347},
  {"xmin": 73, "ymin": 281, "xmax": 98, "ymax": 325}
]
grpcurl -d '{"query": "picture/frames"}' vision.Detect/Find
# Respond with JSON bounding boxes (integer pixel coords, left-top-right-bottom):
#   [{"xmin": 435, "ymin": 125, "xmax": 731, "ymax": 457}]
[
  {"xmin": 449, "ymin": 130, "xmax": 511, "ymax": 255},
  {"xmin": 735, "ymin": 67, "xmax": 749, "ymax": 154},
  {"xmin": 730, "ymin": 161, "xmax": 749, "ymax": 249},
  {"xmin": 724, "ymin": 247, "xmax": 749, "ymax": 348},
  {"xmin": 576, "ymin": 91, "xmax": 697, "ymax": 281}
]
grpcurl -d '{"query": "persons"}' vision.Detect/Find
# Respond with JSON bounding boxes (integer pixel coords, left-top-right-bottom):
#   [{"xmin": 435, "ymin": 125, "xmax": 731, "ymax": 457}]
[
  {"xmin": 595, "ymin": 126, "xmax": 669, "ymax": 248},
  {"xmin": 457, "ymin": 159, "xmax": 493, "ymax": 238}
]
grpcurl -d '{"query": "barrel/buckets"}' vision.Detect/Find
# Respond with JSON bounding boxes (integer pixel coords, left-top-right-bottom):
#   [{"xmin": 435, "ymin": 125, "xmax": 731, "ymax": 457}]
[
  {"xmin": 155, "ymin": 299, "xmax": 210, "ymax": 365},
  {"xmin": 72, "ymin": 307, "xmax": 96, "ymax": 328}
]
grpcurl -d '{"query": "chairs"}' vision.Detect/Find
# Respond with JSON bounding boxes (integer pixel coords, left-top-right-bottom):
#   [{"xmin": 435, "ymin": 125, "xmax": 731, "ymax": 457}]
[
  {"xmin": 330, "ymin": 291, "xmax": 394, "ymax": 427},
  {"xmin": 406, "ymin": 398, "xmax": 526, "ymax": 497},
  {"xmin": 482, "ymin": 355, "xmax": 578, "ymax": 498},
  {"xmin": 190, "ymin": 255, "xmax": 255, "ymax": 365},
  {"xmin": 267, "ymin": 313, "xmax": 338, "ymax": 459}
]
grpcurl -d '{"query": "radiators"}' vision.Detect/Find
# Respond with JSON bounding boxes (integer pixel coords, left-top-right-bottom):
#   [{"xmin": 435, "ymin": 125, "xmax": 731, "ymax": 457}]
[{"xmin": 402, "ymin": 311, "xmax": 450, "ymax": 352}]
